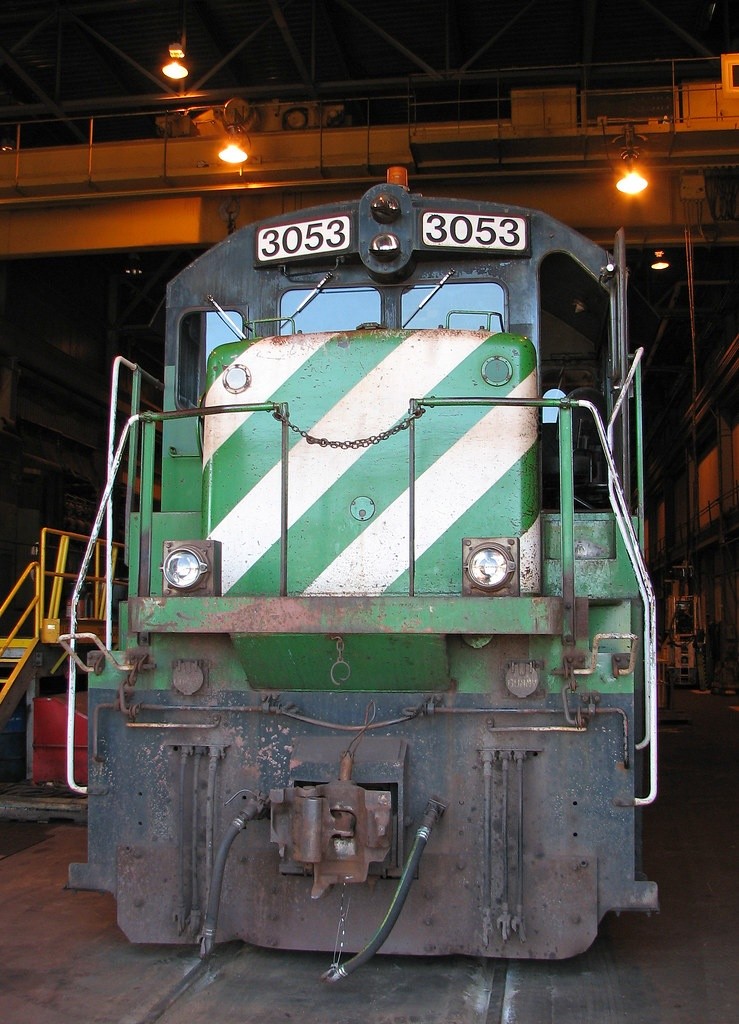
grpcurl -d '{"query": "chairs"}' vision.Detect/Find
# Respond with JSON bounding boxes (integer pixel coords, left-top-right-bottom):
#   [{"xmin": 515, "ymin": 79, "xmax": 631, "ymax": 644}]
[{"xmin": 556, "ymin": 387, "xmax": 607, "ymax": 448}]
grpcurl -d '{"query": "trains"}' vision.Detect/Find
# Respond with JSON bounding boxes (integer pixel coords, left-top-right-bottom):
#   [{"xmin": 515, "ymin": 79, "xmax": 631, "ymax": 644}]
[{"xmin": 66, "ymin": 167, "xmax": 659, "ymax": 978}]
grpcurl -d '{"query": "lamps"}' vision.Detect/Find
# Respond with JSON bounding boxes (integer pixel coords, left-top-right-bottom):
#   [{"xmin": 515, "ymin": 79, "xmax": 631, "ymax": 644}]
[
  {"xmin": 651, "ymin": 250, "xmax": 669, "ymax": 270},
  {"xmin": 613, "ymin": 124, "xmax": 649, "ymax": 195},
  {"xmin": 162, "ymin": 42, "xmax": 189, "ymax": 80},
  {"xmin": 217, "ymin": 124, "xmax": 248, "ymax": 163}
]
